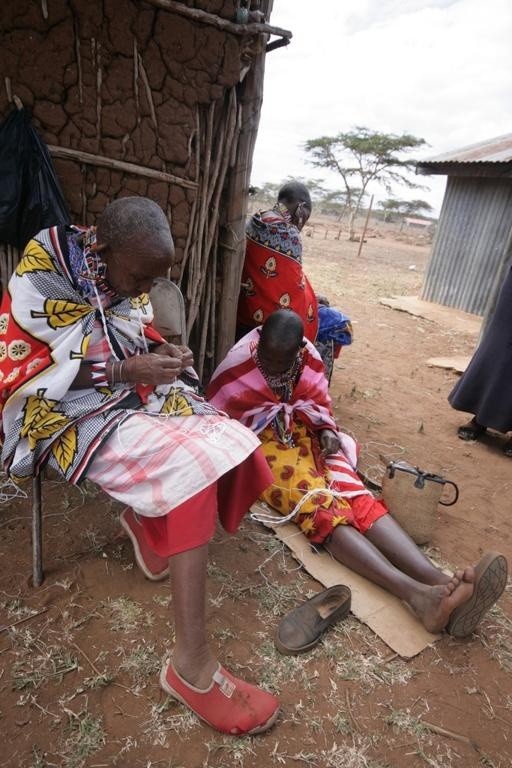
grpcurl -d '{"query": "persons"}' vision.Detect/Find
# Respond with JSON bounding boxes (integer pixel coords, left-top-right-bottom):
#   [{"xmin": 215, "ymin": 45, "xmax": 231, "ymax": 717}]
[
  {"xmin": 235, "ymin": 181, "xmax": 355, "ymax": 388},
  {"xmin": 0, "ymin": 195, "xmax": 283, "ymax": 737},
  {"xmin": 447, "ymin": 229, "xmax": 512, "ymax": 443},
  {"xmin": 202, "ymin": 308, "xmax": 508, "ymax": 639}
]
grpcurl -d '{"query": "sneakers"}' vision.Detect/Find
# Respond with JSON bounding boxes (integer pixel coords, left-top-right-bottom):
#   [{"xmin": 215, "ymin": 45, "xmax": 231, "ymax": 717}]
[
  {"xmin": 445, "ymin": 549, "xmax": 506, "ymax": 640},
  {"xmin": 272, "ymin": 584, "xmax": 352, "ymax": 655},
  {"xmin": 119, "ymin": 506, "xmax": 170, "ymax": 583},
  {"xmin": 455, "ymin": 419, "xmax": 511, "ymax": 457},
  {"xmin": 160, "ymin": 653, "xmax": 282, "ymax": 736}
]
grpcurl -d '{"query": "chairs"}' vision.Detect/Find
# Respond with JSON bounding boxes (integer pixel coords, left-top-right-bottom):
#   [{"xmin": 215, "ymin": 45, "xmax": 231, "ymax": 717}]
[{"xmin": 29, "ymin": 277, "xmax": 191, "ymax": 585}]
